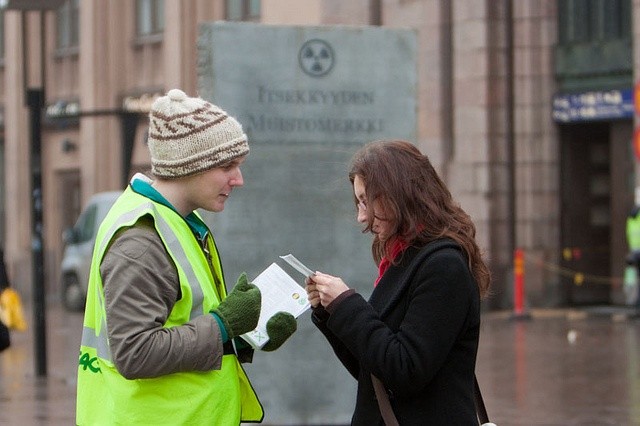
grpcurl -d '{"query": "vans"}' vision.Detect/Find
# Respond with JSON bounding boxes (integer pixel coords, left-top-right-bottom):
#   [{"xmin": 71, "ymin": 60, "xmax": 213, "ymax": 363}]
[{"xmin": 58, "ymin": 192, "xmax": 126, "ymax": 311}]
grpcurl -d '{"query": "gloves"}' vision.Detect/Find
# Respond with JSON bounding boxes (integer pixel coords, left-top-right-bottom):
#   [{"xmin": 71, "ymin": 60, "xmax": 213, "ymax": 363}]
[
  {"xmin": 261, "ymin": 312, "xmax": 297, "ymax": 352},
  {"xmin": 209, "ymin": 272, "xmax": 261, "ymax": 338}
]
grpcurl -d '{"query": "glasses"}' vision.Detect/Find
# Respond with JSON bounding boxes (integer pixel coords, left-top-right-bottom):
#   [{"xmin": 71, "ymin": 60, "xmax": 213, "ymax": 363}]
[{"xmin": 356, "ymin": 196, "xmax": 378, "ymax": 211}]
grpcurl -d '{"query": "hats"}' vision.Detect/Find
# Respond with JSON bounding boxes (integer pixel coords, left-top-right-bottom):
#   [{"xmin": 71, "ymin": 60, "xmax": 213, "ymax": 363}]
[{"xmin": 148, "ymin": 88, "xmax": 250, "ymax": 180}]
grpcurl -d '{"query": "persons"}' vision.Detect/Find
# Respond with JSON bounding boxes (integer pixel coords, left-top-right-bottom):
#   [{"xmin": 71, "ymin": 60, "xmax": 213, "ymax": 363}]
[
  {"xmin": 74, "ymin": 89, "xmax": 298, "ymax": 426},
  {"xmin": 305, "ymin": 140, "xmax": 498, "ymax": 426}
]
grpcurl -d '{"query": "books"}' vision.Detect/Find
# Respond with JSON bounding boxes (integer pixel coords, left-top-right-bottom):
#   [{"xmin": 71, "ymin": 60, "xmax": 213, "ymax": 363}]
[{"xmin": 239, "ymin": 262, "xmax": 311, "ymax": 350}]
